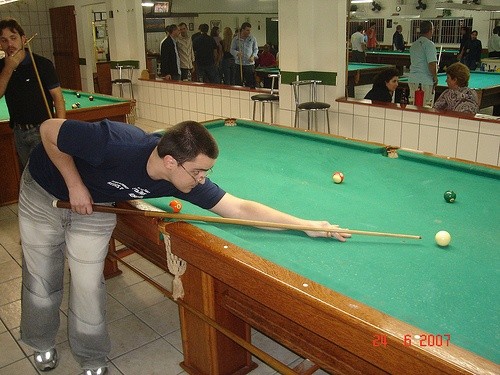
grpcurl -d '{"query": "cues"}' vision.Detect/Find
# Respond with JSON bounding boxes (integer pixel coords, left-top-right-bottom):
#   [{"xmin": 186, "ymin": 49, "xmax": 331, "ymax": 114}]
[
  {"xmin": 26, "ymin": 41, "xmax": 55, "ymax": 120},
  {"xmin": 51, "ymin": 198, "xmax": 422, "ymax": 241},
  {"xmin": 431, "ymin": 45, "xmax": 443, "ymax": 109},
  {"xmin": 237, "ymin": 24, "xmax": 244, "ymax": 85},
  {"xmin": 25, "ymin": 32, "xmax": 40, "ymax": 45}
]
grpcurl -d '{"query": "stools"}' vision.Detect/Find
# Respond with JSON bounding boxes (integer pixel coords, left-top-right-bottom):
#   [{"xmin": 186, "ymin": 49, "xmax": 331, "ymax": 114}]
[
  {"xmin": 290, "ymin": 78, "xmax": 332, "ymax": 134},
  {"xmin": 250, "ymin": 73, "xmax": 281, "ymax": 124},
  {"xmin": 108, "ymin": 64, "xmax": 136, "ymax": 102}
]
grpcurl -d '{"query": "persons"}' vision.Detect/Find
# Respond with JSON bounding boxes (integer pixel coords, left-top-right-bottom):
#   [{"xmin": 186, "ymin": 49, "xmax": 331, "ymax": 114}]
[
  {"xmin": 0, "ymin": 17, "xmax": 65, "ymax": 174},
  {"xmin": 433, "ymin": 62, "xmax": 479, "ymax": 115},
  {"xmin": 408, "ymin": 21, "xmax": 438, "ymax": 107},
  {"xmin": 230, "ymin": 23, "xmax": 259, "ymax": 88},
  {"xmin": 351, "ymin": 25, "xmax": 367, "ymax": 85},
  {"xmin": 457, "ymin": 26, "xmax": 482, "ymax": 70},
  {"xmin": 177, "ymin": 23, "xmax": 195, "ymax": 80},
  {"xmin": 258, "ymin": 45, "xmax": 275, "ymax": 87},
  {"xmin": 393, "ymin": 25, "xmax": 405, "ymax": 50},
  {"xmin": 362, "ymin": 29, "xmax": 368, "ymax": 42},
  {"xmin": 365, "ymin": 69, "xmax": 399, "ymax": 103},
  {"xmin": 20, "ymin": 118, "xmax": 350, "ymax": 375},
  {"xmin": 221, "ymin": 27, "xmax": 236, "ymax": 85},
  {"xmin": 160, "ymin": 24, "xmax": 181, "ymax": 81},
  {"xmin": 191, "ymin": 24, "xmax": 223, "ymax": 83},
  {"xmin": 367, "ymin": 22, "xmax": 379, "ymax": 51},
  {"xmin": 488, "ymin": 26, "xmax": 500, "ymax": 58}
]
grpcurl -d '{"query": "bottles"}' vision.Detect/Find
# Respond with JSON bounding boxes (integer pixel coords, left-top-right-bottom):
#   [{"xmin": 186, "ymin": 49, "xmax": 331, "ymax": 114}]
[
  {"xmin": 415, "ymin": 83, "xmax": 424, "ymax": 105},
  {"xmin": 187, "ymin": 71, "xmax": 191, "ymax": 81},
  {"xmin": 400, "ymin": 88, "xmax": 408, "ymax": 104}
]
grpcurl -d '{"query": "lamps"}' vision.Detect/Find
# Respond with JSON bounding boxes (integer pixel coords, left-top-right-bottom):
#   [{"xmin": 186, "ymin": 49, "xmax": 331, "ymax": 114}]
[{"xmin": 348, "ymin": 0, "xmax": 500, "ymax": 21}]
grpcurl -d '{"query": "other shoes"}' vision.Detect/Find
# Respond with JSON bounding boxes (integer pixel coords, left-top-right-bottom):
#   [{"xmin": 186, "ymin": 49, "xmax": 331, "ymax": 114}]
[{"xmin": 355, "ymin": 80, "xmax": 358, "ymax": 84}]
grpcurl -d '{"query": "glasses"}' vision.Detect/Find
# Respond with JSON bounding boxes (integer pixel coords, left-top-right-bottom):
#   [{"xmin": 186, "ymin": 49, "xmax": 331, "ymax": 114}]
[{"xmin": 174, "ymin": 158, "xmax": 213, "ymax": 187}]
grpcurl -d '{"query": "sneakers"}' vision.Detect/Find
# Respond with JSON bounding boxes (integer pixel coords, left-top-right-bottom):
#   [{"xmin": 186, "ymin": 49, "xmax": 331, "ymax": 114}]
[
  {"xmin": 34, "ymin": 347, "xmax": 59, "ymax": 371},
  {"xmin": 84, "ymin": 366, "xmax": 108, "ymax": 375}
]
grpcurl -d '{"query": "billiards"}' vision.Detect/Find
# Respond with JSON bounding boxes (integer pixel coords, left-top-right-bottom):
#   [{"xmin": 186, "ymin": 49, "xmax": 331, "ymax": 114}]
[
  {"xmin": 169, "ymin": 199, "xmax": 182, "ymax": 213},
  {"xmin": 89, "ymin": 95, "xmax": 94, "ymax": 101},
  {"xmin": 71, "ymin": 102, "xmax": 81, "ymax": 109},
  {"xmin": 332, "ymin": 170, "xmax": 345, "ymax": 184},
  {"xmin": 77, "ymin": 93, "xmax": 80, "ymax": 98},
  {"xmin": 443, "ymin": 190, "xmax": 456, "ymax": 203},
  {"xmin": 444, "ymin": 50, "xmax": 445, "ymax": 51},
  {"xmin": 434, "ymin": 230, "xmax": 452, "ymax": 247}
]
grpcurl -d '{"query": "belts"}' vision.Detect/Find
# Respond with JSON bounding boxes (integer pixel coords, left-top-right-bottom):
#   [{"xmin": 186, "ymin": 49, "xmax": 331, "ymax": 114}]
[{"xmin": 12, "ymin": 122, "xmax": 42, "ymax": 132}]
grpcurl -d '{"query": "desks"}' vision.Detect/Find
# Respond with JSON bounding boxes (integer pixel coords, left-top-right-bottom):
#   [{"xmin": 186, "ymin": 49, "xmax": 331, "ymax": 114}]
[
  {"xmin": 101, "ymin": 115, "xmax": 500, "ymax": 375},
  {"xmin": 252, "ymin": 61, "xmax": 398, "ymax": 98},
  {"xmin": 364, "ymin": 50, "xmax": 442, "ymax": 66},
  {"xmin": 0, "ymin": 87, "xmax": 137, "ymax": 210},
  {"xmin": 394, "ymin": 69, "xmax": 500, "ymax": 116},
  {"xmin": 405, "ymin": 48, "xmax": 460, "ymax": 55}
]
